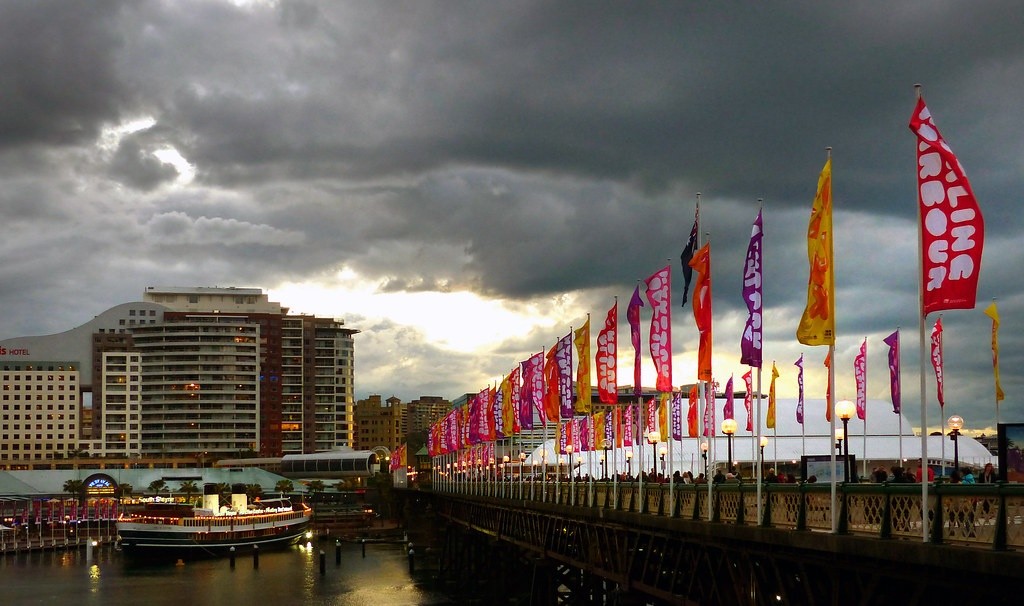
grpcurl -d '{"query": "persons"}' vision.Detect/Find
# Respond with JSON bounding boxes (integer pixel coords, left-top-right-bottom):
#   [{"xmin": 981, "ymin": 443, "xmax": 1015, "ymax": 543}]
[
  {"xmin": 765, "ymin": 467, "xmax": 799, "ymax": 521},
  {"xmin": 713, "ymin": 465, "xmax": 743, "ymax": 517},
  {"xmin": 870, "ymin": 457, "xmax": 996, "ymax": 538},
  {"xmin": 504, "ymin": 470, "xmax": 629, "ymax": 482},
  {"xmin": 673, "ymin": 471, "xmax": 708, "ymax": 514},
  {"xmin": 635, "ymin": 468, "xmax": 670, "ymax": 483}
]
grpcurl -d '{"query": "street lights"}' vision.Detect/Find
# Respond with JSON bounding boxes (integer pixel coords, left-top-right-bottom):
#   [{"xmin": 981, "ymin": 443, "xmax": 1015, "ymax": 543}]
[
  {"xmin": 659, "ymin": 446, "xmax": 667, "ymax": 482},
  {"xmin": 626, "ymin": 451, "xmax": 633, "ymax": 482},
  {"xmin": 577, "ymin": 457, "xmax": 583, "ymax": 482},
  {"xmin": 503, "ymin": 455, "xmax": 509, "ymax": 482},
  {"xmin": 518, "ymin": 453, "xmax": 526, "ymax": 481},
  {"xmin": 834, "ymin": 428, "xmax": 844, "ymax": 455},
  {"xmin": 721, "ymin": 418, "xmax": 738, "ymax": 473},
  {"xmin": 565, "ymin": 445, "xmax": 574, "ymax": 482},
  {"xmin": 489, "ymin": 457, "xmax": 494, "ymax": 481},
  {"xmin": 600, "ymin": 439, "xmax": 612, "ymax": 482},
  {"xmin": 834, "ymin": 399, "xmax": 856, "ymax": 483},
  {"xmin": 701, "ymin": 442, "xmax": 708, "ymax": 480},
  {"xmin": 558, "ymin": 458, "xmax": 564, "ymax": 481},
  {"xmin": 647, "ymin": 431, "xmax": 661, "ymax": 483},
  {"xmin": 946, "ymin": 415, "xmax": 964, "ymax": 482},
  {"xmin": 540, "ymin": 449, "xmax": 547, "ymax": 481},
  {"xmin": 434, "ymin": 459, "xmax": 482, "ymax": 482},
  {"xmin": 600, "ymin": 455, "xmax": 605, "ymax": 480},
  {"xmin": 759, "ymin": 436, "xmax": 769, "ymax": 481}
]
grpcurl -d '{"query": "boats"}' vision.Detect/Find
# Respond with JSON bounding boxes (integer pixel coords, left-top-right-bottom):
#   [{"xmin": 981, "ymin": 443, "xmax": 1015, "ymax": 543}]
[{"xmin": 115, "ymin": 501, "xmax": 311, "ymax": 557}]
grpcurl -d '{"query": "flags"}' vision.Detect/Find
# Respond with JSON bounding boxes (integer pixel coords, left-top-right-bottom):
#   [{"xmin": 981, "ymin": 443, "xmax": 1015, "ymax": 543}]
[
  {"xmin": 740, "ymin": 212, "xmax": 765, "ymax": 367},
  {"xmin": 983, "ymin": 303, "xmax": 1004, "ymax": 400},
  {"xmin": 11, "ymin": 500, "xmax": 118, "ymax": 526},
  {"xmin": 554, "ymin": 351, "xmax": 831, "ymax": 455},
  {"xmin": 853, "ymin": 340, "xmax": 865, "ymax": 420},
  {"xmin": 689, "ymin": 242, "xmax": 712, "ymax": 383},
  {"xmin": 681, "ymin": 205, "xmax": 698, "ymax": 308},
  {"xmin": 883, "ymin": 331, "xmax": 901, "ymax": 414},
  {"xmin": 457, "ymin": 443, "xmax": 494, "ymax": 471},
  {"xmin": 428, "ymin": 265, "xmax": 670, "ymax": 455},
  {"xmin": 930, "ymin": 318, "xmax": 944, "ymax": 407},
  {"xmin": 390, "ymin": 444, "xmax": 407, "ymax": 471},
  {"xmin": 909, "ymin": 96, "xmax": 985, "ymax": 318},
  {"xmin": 797, "ymin": 160, "xmax": 834, "ymax": 348}
]
